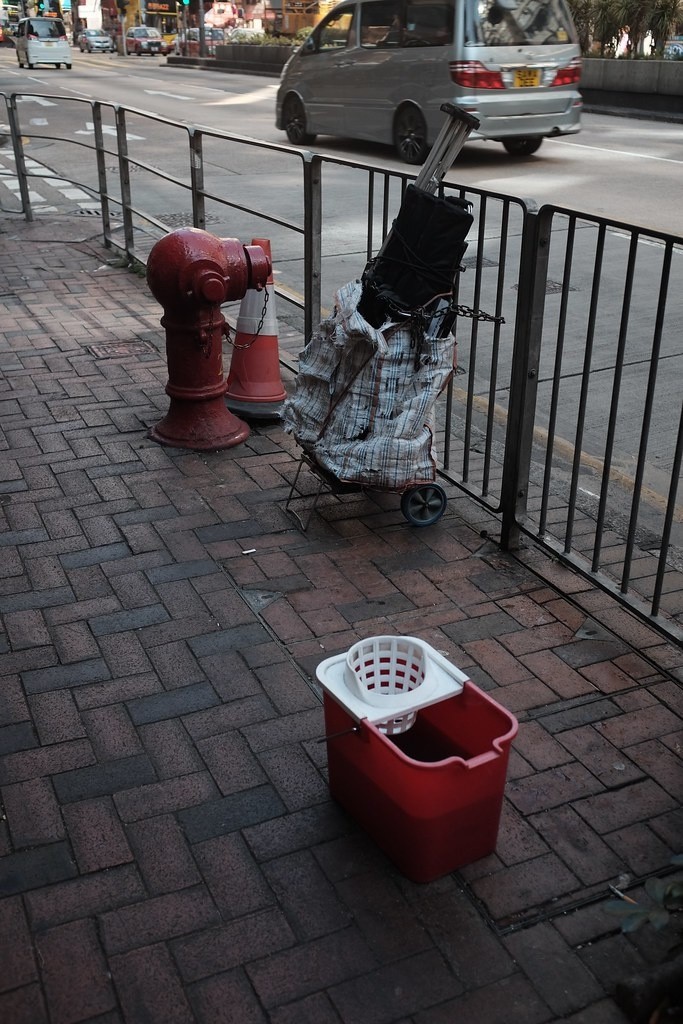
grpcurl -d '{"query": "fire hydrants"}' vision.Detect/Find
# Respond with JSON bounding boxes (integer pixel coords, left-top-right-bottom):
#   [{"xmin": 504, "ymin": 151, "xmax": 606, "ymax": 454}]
[{"xmin": 146, "ymin": 226, "xmax": 272, "ymax": 450}]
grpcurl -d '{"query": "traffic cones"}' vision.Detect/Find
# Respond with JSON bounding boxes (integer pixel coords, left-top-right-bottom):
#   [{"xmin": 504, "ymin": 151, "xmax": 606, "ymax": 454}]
[{"xmin": 223, "ymin": 238, "xmax": 289, "ymax": 423}]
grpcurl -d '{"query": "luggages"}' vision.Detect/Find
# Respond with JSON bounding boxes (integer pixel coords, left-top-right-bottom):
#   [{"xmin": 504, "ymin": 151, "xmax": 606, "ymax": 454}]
[{"xmin": 279, "ymin": 102, "xmax": 482, "ymax": 533}]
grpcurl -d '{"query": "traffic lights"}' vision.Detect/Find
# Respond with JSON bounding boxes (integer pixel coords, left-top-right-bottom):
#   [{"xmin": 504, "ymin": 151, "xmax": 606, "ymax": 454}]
[
  {"xmin": 182, "ymin": 0, "xmax": 189, "ymax": 5},
  {"xmin": 37, "ymin": 1, "xmax": 45, "ymax": 10}
]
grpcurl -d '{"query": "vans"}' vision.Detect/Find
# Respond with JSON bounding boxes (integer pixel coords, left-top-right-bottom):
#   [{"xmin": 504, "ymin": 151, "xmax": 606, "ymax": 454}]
[
  {"xmin": 125, "ymin": 27, "xmax": 170, "ymax": 56},
  {"xmin": 78, "ymin": 29, "xmax": 115, "ymax": 53},
  {"xmin": 274, "ymin": 0, "xmax": 583, "ymax": 165},
  {"xmin": 13, "ymin": 17, "xmax": 72, "ymax": 69}
]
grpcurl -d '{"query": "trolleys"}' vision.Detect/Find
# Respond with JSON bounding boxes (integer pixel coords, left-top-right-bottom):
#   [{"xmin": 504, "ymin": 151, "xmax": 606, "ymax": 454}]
[{"xmin": 282, "ymin": 102, "xmax": 484, "ymax": 534}]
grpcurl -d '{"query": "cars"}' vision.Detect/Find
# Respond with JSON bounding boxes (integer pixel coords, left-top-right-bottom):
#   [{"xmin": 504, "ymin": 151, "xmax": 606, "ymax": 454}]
[
  {"xmin": 176, "ymin": 27, "xmax": 225, "ymax": 58},
  {"xmin": 226, "ymin": 28, "xmax": 266, "ymax": 45},
  {"xmin": 162, "ymin": 28, "xmax": 179, "ymax": 51}
]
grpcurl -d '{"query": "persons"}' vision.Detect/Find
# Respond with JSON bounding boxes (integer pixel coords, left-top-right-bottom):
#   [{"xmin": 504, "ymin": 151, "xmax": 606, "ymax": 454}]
[
  {"xmin": 110, "ymin": 27, "xmax": 119, "ymax": 53},
  {"xmin": 603, "ymin": 24, "xmax": 656, "ymax": 58},
  {"xmin": 0, "ymin": 19, "xmax": 17, "ymax": 48}
]
